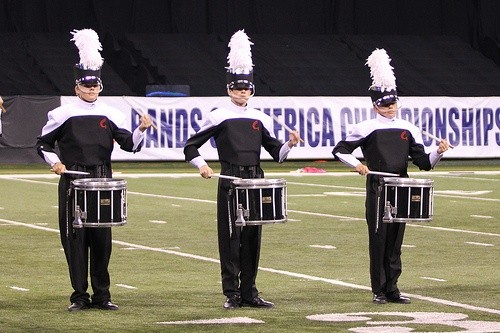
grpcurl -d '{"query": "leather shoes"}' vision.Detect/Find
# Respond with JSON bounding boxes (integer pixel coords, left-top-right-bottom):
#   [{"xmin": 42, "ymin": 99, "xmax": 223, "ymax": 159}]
[
  {"xmin": 223, "ymin": 295, "xmax": 243, "ymax": 309},
  {"xmin": 68, "ymin": 299, "xmax": 91, "ymax": 311},
  {"xmin": 242, "ymin": 292, "xmax": 275, "ymax": 309},
  {"xmin": 385, "ymin": 292, "xmax": 411, "ymax": 304},
  {"xmin": 90, "ymin": 298, "xmax": 119, "ymax": 310},
  {"xmin": 373, "ymin": 292, "xmax": 387, "ymax": 304}
]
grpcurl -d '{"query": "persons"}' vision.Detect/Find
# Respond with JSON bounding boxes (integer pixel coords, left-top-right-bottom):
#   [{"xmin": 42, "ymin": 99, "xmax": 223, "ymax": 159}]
[
  {"xmin": 332, "ymin": 85, "xmax": 449, "ymax": 304},
  {"xmin": 183, "ymin": 67, "xmax": 299, "ymax": 310},
  {"xmin": 0, "ymin": 96, "xmax": 6, "ymax": 134},
  {"xmin": 36, "ymin": 65, "xmax": 153, "ymax": 311}
]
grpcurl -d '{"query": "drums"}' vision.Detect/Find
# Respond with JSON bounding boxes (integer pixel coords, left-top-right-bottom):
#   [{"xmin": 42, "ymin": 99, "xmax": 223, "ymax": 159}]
[
  {"xmin": 229, "ymin": 177, "xmax": 288, "ymax": 227},
  {"xmin": 377, "ymin": 176, "xmax": 434, "ymax": 224},
  {"xmin": 69, "ymin": 174, "xmax": 130, "ymax": 232}
]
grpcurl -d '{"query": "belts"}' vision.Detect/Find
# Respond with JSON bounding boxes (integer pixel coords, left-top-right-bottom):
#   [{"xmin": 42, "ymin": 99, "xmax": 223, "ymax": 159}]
[
  {"xmin": 373, "ymin": 169, "xmax": 407, "ymax": 180},
  {"xmin": 70, "ymin": 166, "xmax": 110, "ymax": 178},
  {"xmin": 221, "ymin": 162, "xmax": 261, "ymax": 179}
]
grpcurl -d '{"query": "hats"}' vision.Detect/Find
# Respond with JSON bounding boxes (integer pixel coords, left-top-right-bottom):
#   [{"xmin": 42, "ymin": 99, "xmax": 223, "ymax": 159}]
[
  {"xmin": 73, "ymin": 63, "xmax": 103, "ymax": 87},
  {"xmin": 225, "ymin": 67, "xmax": 254, "ymax": 90},
  {"xmin": 368, "ymin": 85, "xmax": 398, "ymax": 107}
]
